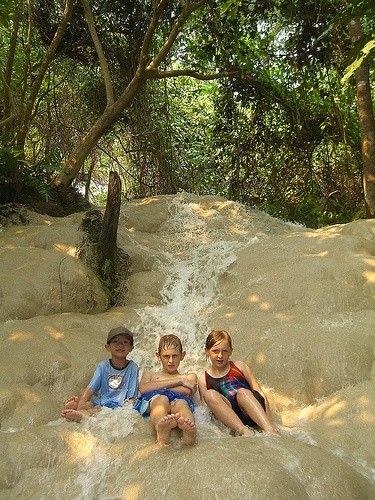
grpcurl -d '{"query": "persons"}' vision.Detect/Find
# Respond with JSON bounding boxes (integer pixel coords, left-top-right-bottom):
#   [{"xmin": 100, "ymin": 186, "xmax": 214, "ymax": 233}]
[
  {"xmin": 198, "ymin": 329, "xmax": 281, "ymax": 438},
  {"xmin": 134, "ymin": 334, "xmax": 198, "ymax": 446},
  {"xmin": 61, "ymin": 326, "xmax": 139, "ymax": 425}
]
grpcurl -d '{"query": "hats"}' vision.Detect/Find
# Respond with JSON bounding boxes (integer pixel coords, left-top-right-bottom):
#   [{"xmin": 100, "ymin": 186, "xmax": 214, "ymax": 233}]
[{"xmin": 106, "ymin": 326, "xmax": 134, "ymax": 345}]
